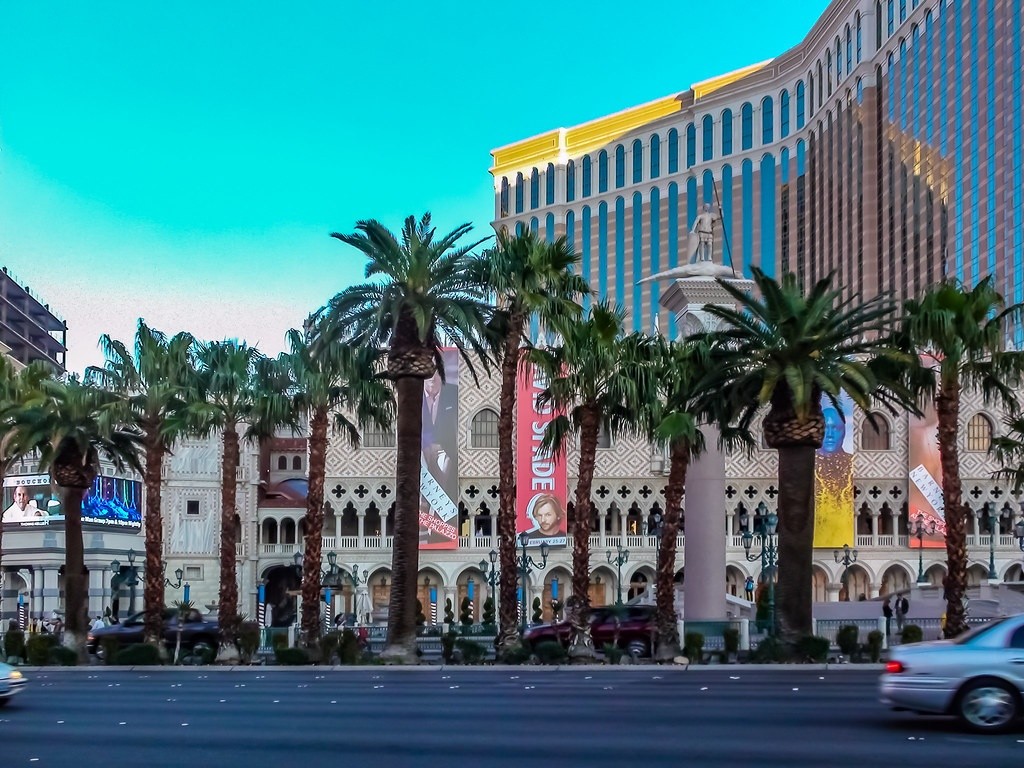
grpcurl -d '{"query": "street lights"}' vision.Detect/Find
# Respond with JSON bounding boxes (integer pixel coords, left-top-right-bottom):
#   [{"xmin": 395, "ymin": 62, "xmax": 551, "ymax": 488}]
[
  {"xmin": 315, "ymin": 551, "xmax": 344, "ymax": 633},
  {"xmin": 834, "ymin": 544, "xmax": 858, "ymax": 601},
  {"xmin": 906, "ymin": 513, "xmax": 936, "ymax": 581},
  {"xmin": 741, "ymin": 499, "xmax": 778, "ymax": 639},
  {"xmin": 977, "ymin": 501, "xmax": 1012, "ymax": 580},
  {"xmin": 606, "ymin": 544, "xmax": 631, "ymax": 604},
  {"xmin": 480, "ymin": 549, "xmax": 502, "ymax": 634},
  {"xmin": 642, "ymin": 513, "xmax": 664, "ymax": 584},
  {"xmin": 114, "ymin": 552, "xmax": 146, "ymax": 623},
  {"xmin": 346, "ymin": 565, "xmax": 369, "ymax": 626},
  {"xmin": 514, "ymin": 531, "xmax": 551, "ymax": 630}
]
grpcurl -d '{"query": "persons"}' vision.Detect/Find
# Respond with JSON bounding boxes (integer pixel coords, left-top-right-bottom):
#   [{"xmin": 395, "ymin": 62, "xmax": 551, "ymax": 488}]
[
  {"xmin": 690, "ymin": 202, "xmax": 722, "ymax": 262},
  {"xmin": 29, "ymin": 615, "xmax": 64, "ymax": 633},
  {"xmin": 894, "ymin": 593, "xmax": 909, "ymax": 635},
  {"xmin": 883, "ymin": 598, "xmax": 893, "ymax": 636},
  {"xmin": 90, "ymin": 616, "xmax": 105, "ymax": 630},
  {"xmin": 744, "ymin": 576, "xmax": 754, "ymax": 602},
  {"xmin": 422, "ymin": 358, "xmax": 457, "ymax": 479},
  {"xmin": 814, "ymin": 408, "xmax": 852, "ymax": 466},
  {"xmin": 1, "ymin": 486, "xmax": 50, "ymax": 518},
  {"xmin": 518, "ymin": 492, "xmax": 566, "ymax": 538}
]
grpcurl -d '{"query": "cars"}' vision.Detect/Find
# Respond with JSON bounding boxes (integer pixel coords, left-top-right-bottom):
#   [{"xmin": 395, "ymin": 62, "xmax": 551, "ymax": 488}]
[
  {"xmin": 0, "ymin": 661, "xmax": 27, "ymax": 705},
  {"xmin": 877, "ymin": 611, "xmax": 1024, "ymax": 734}
]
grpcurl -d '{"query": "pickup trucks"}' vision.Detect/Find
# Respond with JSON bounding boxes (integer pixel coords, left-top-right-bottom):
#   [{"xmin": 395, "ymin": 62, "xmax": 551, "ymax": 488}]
[{"xmin": 87, "ymin": 606, "xmax": 260, "ymax": 665}]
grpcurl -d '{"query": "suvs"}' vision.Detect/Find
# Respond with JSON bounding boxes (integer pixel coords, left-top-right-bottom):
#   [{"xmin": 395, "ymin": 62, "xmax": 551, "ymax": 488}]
[{"xmin": 520, "ymin": 601, "xmax": 658, "ymax": 660}]
